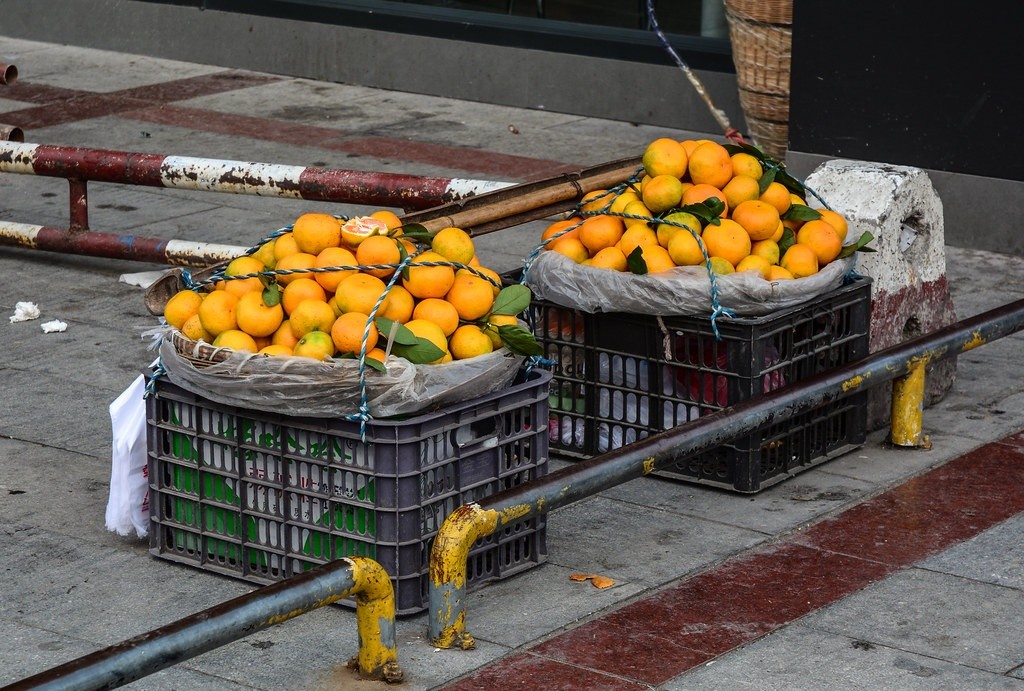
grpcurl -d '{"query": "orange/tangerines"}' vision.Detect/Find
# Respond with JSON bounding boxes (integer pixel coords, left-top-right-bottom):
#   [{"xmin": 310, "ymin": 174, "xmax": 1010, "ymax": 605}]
[
  {"xmin": 542, "ymin": 138, "xmax": 879, "ymax": 281},
  {"xmin": 162, "ymin": 213, "xmax": 542, "ymax": 375}
]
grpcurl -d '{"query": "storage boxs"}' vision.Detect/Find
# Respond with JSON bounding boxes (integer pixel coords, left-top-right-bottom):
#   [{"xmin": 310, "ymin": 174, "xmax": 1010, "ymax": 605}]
[
  {"xmin": 142, "ymin": 366, "xmax": 554, "ymax": 617},
  {"xmin": 515, "ymin": 274, "xmax": 874, "ymax": 496}
]
